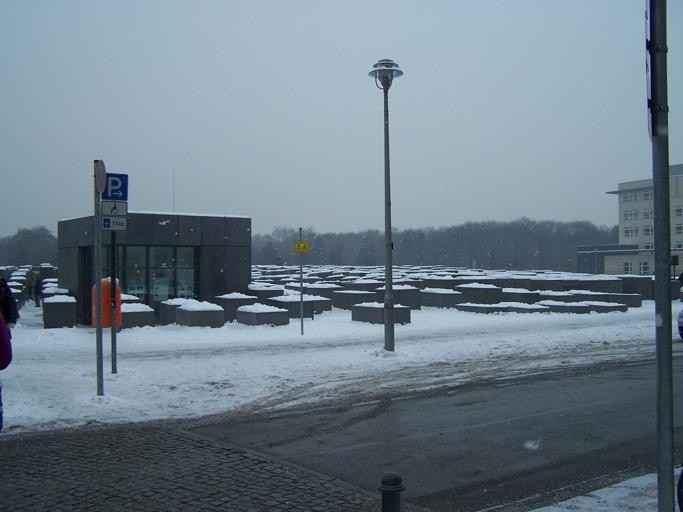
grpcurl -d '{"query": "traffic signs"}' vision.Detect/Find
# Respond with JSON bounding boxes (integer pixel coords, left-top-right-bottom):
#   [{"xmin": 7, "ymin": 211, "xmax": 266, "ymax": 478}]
[
  {"xmin": 100, "ymin": 201, "xmax": 127, "ymax": 216},
  {"xmin": 295, "ymin": 239, "xmax": 310, "ymax": 253},
  {"xmin": 101, "ymin": 172, "xmax": 129, "ymax": 201},
  {"xmin": 100, "ymin": 216, "xmax": 125, "ymax": 233}
]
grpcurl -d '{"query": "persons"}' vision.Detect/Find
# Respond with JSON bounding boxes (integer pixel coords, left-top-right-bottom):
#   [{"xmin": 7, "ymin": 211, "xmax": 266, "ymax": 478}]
[
  {"xmin": 0, "ymin": 276, "xmax": 17, "ymax": 430},
  {"xmin": 31, "ymin": 270, "xmax": 40, "ymax": 306}
]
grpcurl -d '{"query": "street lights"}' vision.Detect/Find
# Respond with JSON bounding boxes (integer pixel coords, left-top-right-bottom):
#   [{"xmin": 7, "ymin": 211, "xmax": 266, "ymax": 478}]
[{"xmin": 367, "ymin": 55, "xmax": 405, "ymax": 353}]
